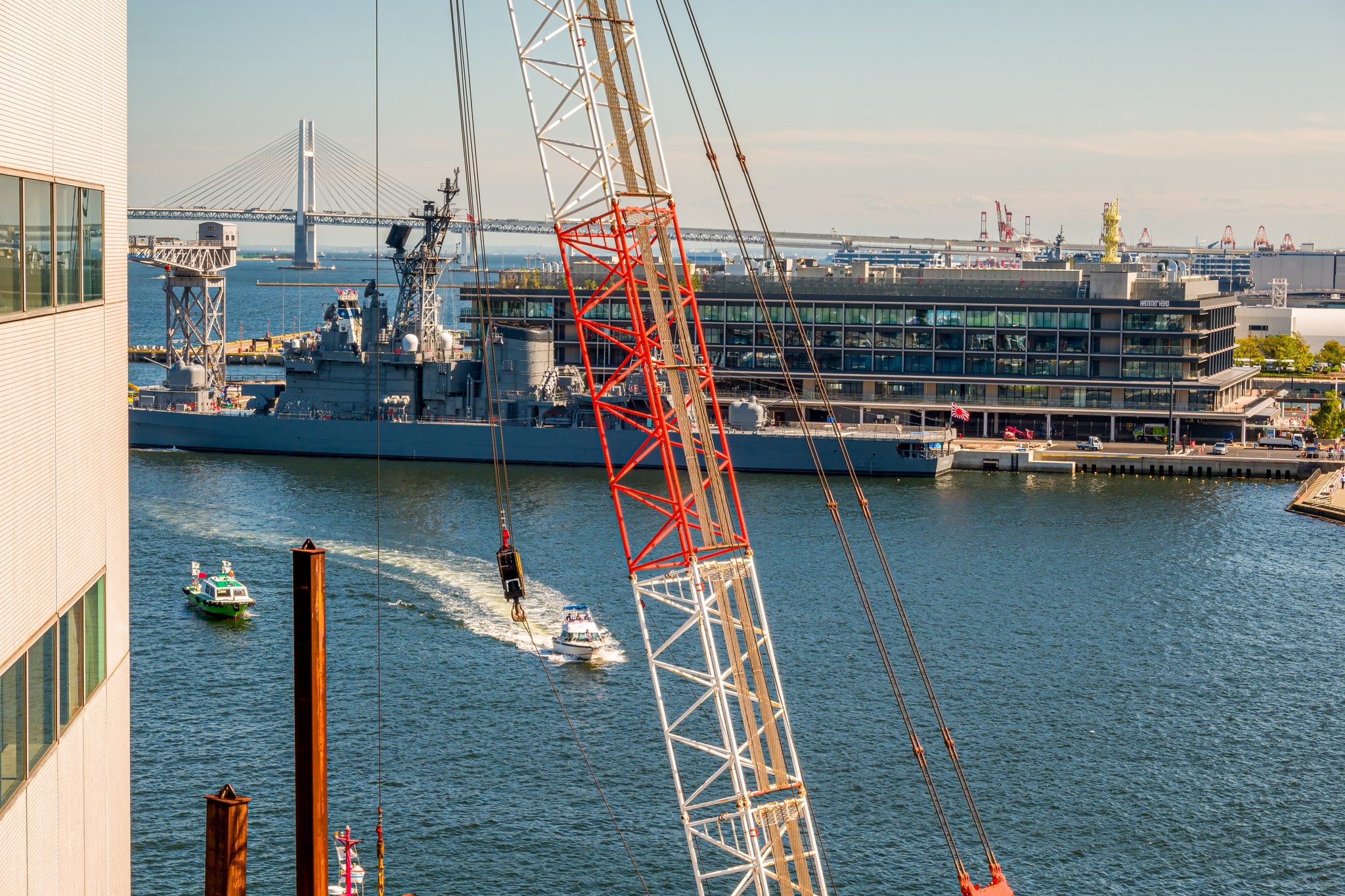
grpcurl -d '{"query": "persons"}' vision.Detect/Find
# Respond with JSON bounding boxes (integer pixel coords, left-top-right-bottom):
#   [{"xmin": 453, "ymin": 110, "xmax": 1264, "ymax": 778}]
[
  {"xmin": 1342, "ymin": 467, "xmax": 1345, "ymax": 478},
  {"xmin": 565, "ymin": 613, "xmax": 572, "ymax": 623},
  {"xmin": 1339, "ymin": 448, "xmax": 1345, "ymax": 460},
  {"xmin": 574, "ymin": 613, "xmax": 581, "ymax": 620},
  {"xmin": 1341, "ymin": 475, "xmax": 1345, "ymax": 489},
  {"xmin": 584, "ymin": 629, "xmax": 593, "ymax": 641},
  {"xmin": 584, "ymin": 611, "xmax": 591, "ymax": 620}
]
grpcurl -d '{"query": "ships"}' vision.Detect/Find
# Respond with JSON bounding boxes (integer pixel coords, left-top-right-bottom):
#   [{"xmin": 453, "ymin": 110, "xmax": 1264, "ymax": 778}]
[{"xmin": 129, "ymin": 165, "xmax": 959, "ymax": 481}]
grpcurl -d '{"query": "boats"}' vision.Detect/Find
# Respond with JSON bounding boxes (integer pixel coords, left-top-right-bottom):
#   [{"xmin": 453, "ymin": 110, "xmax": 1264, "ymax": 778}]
[
  {"xmin": 182, "ymin": 560, "xmax": 256, "ymax": 621},
  {"xmin": 551, "ymin": 604, "xmax": 606, "ymax": 660}
]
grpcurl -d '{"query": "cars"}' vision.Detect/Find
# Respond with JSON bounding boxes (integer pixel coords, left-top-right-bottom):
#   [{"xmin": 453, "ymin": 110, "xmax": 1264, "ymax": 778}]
[
  {"xmin": 1213, "ymin": 442, "xmax": 1228, "ymax": 455},
  {"xmin": 1305, "ymin": 446, "xmax": 1319, "ymax": 457},
  {"xmin": 1242, "ymin": 360, "xmax": 1332, "ymax": 372}
]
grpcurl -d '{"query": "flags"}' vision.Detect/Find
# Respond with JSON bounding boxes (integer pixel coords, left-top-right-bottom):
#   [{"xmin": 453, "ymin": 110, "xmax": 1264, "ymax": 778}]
[{"xmin": 951, "ymin": 403, "xmax": 970, "ymax": 421}]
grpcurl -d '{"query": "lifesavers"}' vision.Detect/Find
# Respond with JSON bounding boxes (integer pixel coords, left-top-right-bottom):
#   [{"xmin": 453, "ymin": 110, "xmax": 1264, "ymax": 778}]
[
  {"xmin": 387, "ymin": 410, "xmax": 393, "ymax": 415},
  {"xmin": 632, "ymin": 387, "xmax": 637, "ymax": 392},
  {"xmin": 189, "ymin": 403, "xmax": 194, "ymax": 409},
  {"xmin": 491, "ymin": 417, "xmax": 497, "ymax": 423},
  {"xmin": 601, "ymin": 423, "xmax": 607, "ymax": 430},
  {"xmin": 395, "ymin": 349, "xmax": 401, "ymax": 354}
]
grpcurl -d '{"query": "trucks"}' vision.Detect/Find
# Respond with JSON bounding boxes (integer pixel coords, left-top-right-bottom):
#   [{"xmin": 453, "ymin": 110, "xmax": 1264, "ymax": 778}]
[
  {"xmin": 1133, "ymin": 423, "xmax": 1168, "ymax": 441},
  {"xmin": 1258, "ymin": 433, "xmax": 1306, "ymax": 450},
  {"xmin": 1076, "ymin": 436, "xmax": 1103, "ymax": 450}
]
